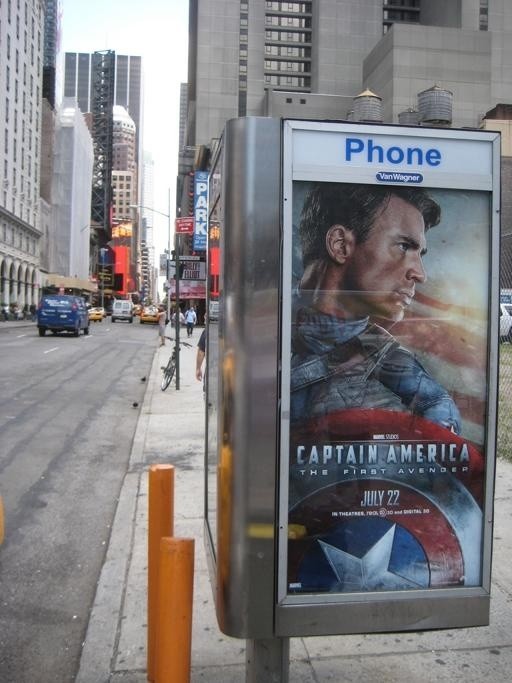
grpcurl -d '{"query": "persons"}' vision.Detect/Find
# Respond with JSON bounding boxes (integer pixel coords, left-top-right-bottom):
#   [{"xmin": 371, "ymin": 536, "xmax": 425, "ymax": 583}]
[
  {"xmin": 289, "ymin": 180, "xmax": 463, "ymax": 488},
  {"xmin": 196, "ymin": 325, "xmax": 206, "ymax": 402},
  {"xmin": 155, "ymin": 304, "xmax": 207, "ymax": 347}
]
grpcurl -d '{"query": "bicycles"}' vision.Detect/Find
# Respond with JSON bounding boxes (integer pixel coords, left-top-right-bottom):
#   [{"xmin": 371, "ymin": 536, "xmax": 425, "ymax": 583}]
[{"xmin": 160, "ymin": 336, "xmax": 193, "ymax": 393}]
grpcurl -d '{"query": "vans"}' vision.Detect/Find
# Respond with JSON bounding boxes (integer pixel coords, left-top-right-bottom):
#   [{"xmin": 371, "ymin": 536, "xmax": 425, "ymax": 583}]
[
  {"xmin": 209, "ymin": 300, "xmax": 220, "ymax": 322},
  {"xmin": 111, "ymin": 300, "xmax": 132, "ymax": 322},
  {"xmin": 37, "ymin": 295, "xmax": 91, "ymax": 337}
]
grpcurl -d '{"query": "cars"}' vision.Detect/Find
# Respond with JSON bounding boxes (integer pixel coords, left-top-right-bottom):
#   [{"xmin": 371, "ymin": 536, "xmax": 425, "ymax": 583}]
[
  {"xmin": 140, "ymin": 305, "xmax": 159, "ymax": 322},
  {"xmin": 88, "ymin": 306, "xmax": 107, "ymax": 321},
  {"xmin": 500, "ymin": 304, "xmax": 512, "ymax": 344}
]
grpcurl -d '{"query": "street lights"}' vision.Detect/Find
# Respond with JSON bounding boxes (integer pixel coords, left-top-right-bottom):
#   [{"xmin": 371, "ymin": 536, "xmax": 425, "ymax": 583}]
[{"xmin": 126, "ymin": 189, "xmax": 175, "ymax": 326}]
